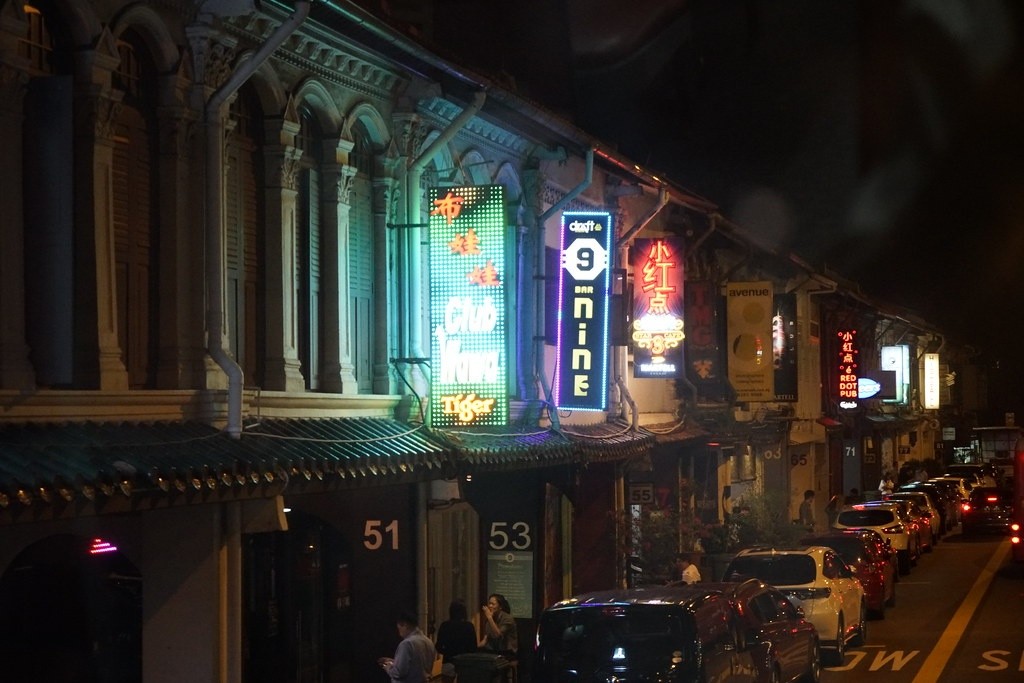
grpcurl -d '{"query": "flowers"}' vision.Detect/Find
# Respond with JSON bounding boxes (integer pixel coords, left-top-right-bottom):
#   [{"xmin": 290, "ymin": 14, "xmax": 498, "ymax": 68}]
[
  {"xmin": 672, "ymin": 475, "xmax": 713, "ymax": 553},
  {"xmin": 616, "ymin": 485, "xmax": 699, "ymax": 576}
]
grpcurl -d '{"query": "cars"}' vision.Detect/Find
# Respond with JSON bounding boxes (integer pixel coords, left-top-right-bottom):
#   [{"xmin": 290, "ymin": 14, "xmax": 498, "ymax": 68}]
[
  {"xmin": 960, "ymin": 485, "xmax": 1014, "ymax": 536},
  {"xmin": 830, "ymin": 474, "xmax": 975, "ymax": 578},
  {"xmin": 721, "ymin": 544, "xmax": 868, "ymax": 666}
]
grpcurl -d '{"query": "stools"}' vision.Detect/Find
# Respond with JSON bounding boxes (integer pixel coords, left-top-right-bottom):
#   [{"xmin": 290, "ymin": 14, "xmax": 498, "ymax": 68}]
[{"xmin": 499, "ymin": 660, "xmax": 520, "ymax": 683}]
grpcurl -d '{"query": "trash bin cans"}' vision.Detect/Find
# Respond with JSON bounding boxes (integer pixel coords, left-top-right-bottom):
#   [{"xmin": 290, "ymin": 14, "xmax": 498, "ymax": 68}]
[{"xmin": 451, "ymin": 652, "xmax": 511, "ymax": 683}]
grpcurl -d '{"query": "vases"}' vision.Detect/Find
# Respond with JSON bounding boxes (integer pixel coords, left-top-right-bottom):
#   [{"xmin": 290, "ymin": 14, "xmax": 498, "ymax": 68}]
[
  {"xmin": 679, "ymin": 548, "xmax": 701, "ymax": 569},
  {"xmin": 634, "ymin": 572, "xmax": 678, "ymax": 588}
]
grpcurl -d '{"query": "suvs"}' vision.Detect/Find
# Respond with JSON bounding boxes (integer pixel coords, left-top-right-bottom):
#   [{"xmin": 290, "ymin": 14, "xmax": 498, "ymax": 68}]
[
  {"xmin": 802, "ymin": 529, "xmax": 899, "ymax": 615},
  {"xmin": 943, "ymin": 463, "xmax": 997, "ymax": 489},
  {"xmin": 532, "ymin": 578, "xmax": 822, "ymax": 682}
]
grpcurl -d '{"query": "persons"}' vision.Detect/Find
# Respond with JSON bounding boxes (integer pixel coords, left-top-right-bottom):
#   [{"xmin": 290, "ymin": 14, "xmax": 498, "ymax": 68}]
[
  {"xmin": 378, "ymin": 610, "xmax": 436, "ymax": 683},
  {"xmin": 842, "ymin": 488, "xmax": 859, "ymax": 505},
  {"xmin": 890, "ymin": 461, "xmax": 898, "ymax": 483},
  {"xmin": 435, "ymin": 599, "xmax": 477, "ymax": 683},
  {"xmin": 799, "ymin": 490, "xmax": 815, "ymax": 533},
  {"xmin": 676, "ymin": 553, "xmax": 701, "ymax": 585},
  {"xmin": 878, "ymin": 472, "xmax": 894, "ymax": 494},
  {"xmin": 824, "ymin": 492, "xmax": 840, "ymax": 532},
  {"xmin": 477, "ymin": 594, "xmax": 519, "ymax": 683}
]
